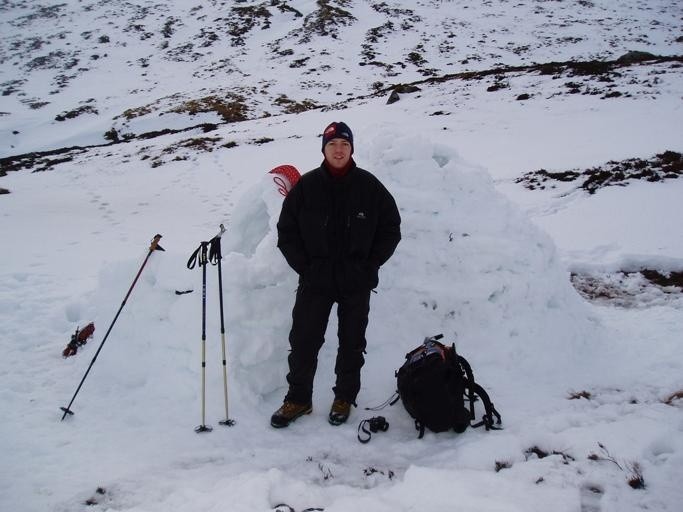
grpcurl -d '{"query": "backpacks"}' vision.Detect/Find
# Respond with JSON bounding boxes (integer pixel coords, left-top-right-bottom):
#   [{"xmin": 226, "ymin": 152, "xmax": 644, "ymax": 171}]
[{"xmin": 395, "ymin": 333, "xmax": 504, "ymax": 433}]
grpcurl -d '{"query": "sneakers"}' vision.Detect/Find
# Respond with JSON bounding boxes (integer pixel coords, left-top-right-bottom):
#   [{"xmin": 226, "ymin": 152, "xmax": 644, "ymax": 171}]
[
  {"xmin": 270, "ymin": 398, "xmax": 312, "ymax": 428},
  {"xmin": 327, "ymin": 395, "xmax": 350, "ymax": 426}
]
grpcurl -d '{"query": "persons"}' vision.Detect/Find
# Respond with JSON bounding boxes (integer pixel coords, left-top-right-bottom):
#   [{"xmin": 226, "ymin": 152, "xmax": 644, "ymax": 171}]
[{"xmin": 266, "ymin": 120, "xmax": 403, "ymax": 432}]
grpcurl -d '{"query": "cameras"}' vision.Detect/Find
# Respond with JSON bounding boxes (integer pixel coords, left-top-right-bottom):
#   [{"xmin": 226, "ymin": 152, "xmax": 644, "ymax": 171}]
[{"xmin": 370, "ymin": 415, "xmax": 388, "ymax": 433}]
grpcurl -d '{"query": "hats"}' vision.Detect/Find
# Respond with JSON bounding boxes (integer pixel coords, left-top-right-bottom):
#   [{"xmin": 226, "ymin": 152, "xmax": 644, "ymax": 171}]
[{"xmin": 321, "ymin": 122, "xmax": 354, "ymax": 154}]
